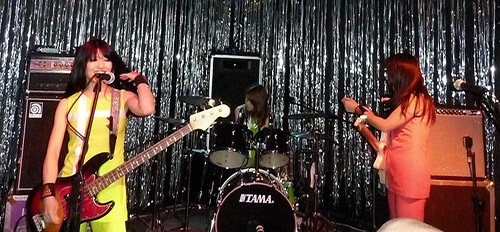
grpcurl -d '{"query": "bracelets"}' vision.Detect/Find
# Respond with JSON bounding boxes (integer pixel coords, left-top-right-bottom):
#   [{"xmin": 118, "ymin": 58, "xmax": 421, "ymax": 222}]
[
  {"xmin": 136, "ymin": 75, "xmax": 149, "ymax": 86},
  {"xmin": 41, "ymin": 183, "xmax": 56, "ymax": 199},
  {"xmin": 356, "ymin": 105, "xmax": 368, "ymax": 118}
]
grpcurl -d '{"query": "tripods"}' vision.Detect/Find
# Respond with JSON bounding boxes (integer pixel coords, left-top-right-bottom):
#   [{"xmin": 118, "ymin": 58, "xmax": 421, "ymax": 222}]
[
  {"xmin": 296, "ymin": 101, "xmax": 337, "ymax": 232},
  {"xmin": 129, "ymin": 125, "xmax": 185, "ymax": 232}
]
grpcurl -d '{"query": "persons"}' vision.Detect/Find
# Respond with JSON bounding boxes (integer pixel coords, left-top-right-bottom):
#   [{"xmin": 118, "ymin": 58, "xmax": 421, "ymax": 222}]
[
  {"xmin": 340, "ymin": 53, "xmax": 436, "ymax": 224},
  {"xmin": 234, "ymin": 82, "xmax": 274, "ymax": 168},
  {"xmin": 42, "ymin": 38, "xmax": 156, "ymax": 232}
]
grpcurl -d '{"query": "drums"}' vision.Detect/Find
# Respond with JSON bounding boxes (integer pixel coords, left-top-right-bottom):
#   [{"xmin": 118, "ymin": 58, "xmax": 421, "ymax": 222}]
[
  {"xmin": 208, "ymin": 123, "xmax": 254, "ymax": 169},
  {"xmin": 213, "ymin": 167, "xmax": 298, "ymax": 232},
  {"xmin": 254, "ymin": 127, "xmax": 290, "ymax": 169}
]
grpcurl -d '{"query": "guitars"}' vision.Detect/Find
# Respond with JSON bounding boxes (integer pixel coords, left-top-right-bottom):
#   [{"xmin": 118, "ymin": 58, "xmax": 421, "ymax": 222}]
[
  {"xmin": 24, "ymin": 103, "xmax": 231, "ymax": 232},
  {"xmin": 354, "ymin": 117, "xmax": 389, "ymax": 189}
]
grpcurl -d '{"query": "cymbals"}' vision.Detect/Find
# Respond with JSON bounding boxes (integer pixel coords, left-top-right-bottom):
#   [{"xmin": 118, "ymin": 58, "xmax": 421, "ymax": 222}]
[
  {"xmin": 153, "ymin": 117, "xmax": 189, "ymax": 124},
  {"xmin": 178, "ymin": 96, "xmax": 218, "ymax": 107}
]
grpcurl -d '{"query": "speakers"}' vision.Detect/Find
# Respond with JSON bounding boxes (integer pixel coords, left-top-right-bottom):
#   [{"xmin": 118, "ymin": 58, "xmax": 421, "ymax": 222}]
[
  {"xmin": 423, "ymin": 179, "xmax": 496, "ymax": 232},
  {"xmin": 206, "ymin": 53, "xmax": 263, "ymax": 151},
  {"xmin": 429, "ymin": 104, "xmax": 487, "ymax": 181},
  {"xmin": 15, "ymin": 95, "xmax": 69, "ymax": 195}
]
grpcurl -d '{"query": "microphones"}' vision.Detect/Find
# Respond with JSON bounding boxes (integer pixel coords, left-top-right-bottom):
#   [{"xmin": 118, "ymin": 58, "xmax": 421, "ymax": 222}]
[
  {"xmin": 462, "ymin": 136, "xmax": 473, "ymax": 164},
  {"xmin": 255, "ymin": 224, "xmax": 264, "ymax": 232},
  {"xmin": 289, "ymin": 97, "xmax": 300, "ymax": 105},
  {"xmin": 95, "ymin": 71, "xmax": 116, "ymax": 84},
  {"xmin": 453, "ymin": 79, "xmax": 488, "ymax": 93}
]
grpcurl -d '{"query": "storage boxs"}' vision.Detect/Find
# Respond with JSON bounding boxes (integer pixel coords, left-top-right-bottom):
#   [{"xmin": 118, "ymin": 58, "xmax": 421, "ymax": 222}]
[{"xmin": 424, "ymin": 175, "xmax": 495, "ymax": 232}]
